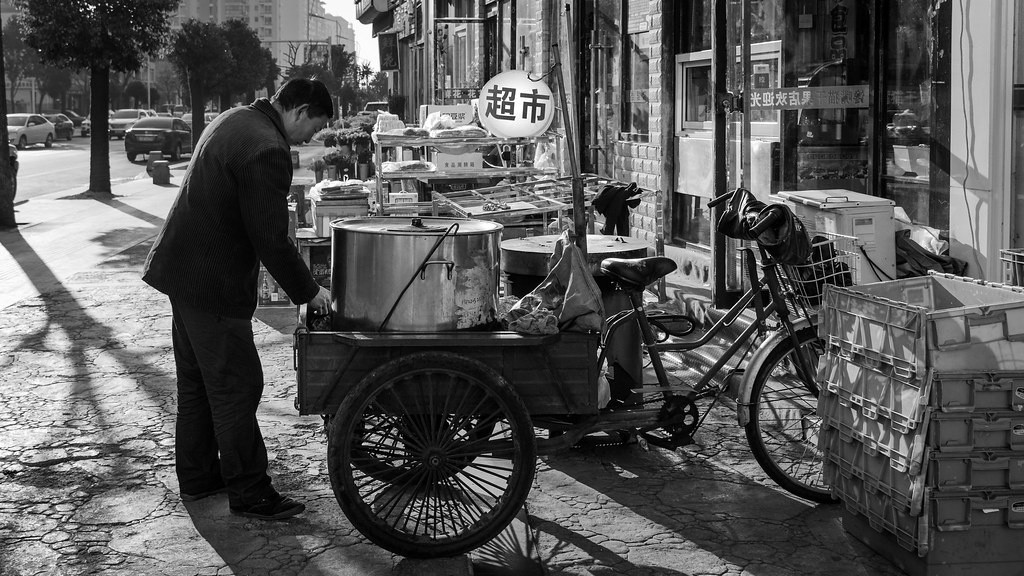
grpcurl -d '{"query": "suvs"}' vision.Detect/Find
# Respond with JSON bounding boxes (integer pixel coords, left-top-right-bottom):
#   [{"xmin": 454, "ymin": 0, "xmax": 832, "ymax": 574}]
[{"xmin": 81, "ymin": 109, "xmax": 117, "ymax": 138}]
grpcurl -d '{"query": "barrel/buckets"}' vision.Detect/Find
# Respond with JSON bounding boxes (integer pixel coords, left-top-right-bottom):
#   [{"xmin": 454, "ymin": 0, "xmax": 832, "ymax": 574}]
[{"xmin": 500, "ymin": 234, "xmax": 648, "ymax": 409}]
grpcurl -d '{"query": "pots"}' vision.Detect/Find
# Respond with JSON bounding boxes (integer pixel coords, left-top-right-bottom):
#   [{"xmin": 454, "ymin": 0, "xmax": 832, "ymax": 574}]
[{"xmin": 329, "ymin": 215, "xmax": 505, "ymax": 332}]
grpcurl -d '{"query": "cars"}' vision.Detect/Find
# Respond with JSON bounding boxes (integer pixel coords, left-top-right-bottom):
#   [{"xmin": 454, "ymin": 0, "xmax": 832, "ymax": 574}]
[
  {"xmin": 143, "ymin": 108, "xmax": 220, "ymax": 142},
  {"xmin": 124, "ymin": 117, "xmax": 192, "ymax": 162},
  {"xmin": 42, "ymin": 113, "xmax": 75, "ymax": 140},
  {"xmin": 108, "ymin": 108, "xmax": 150, "ymax": 140},
  {"xmin": 64, "ymin": 110, "xmax": 85, "ymax": 125},
  {"xmin": 6, "ymin": 113, "xmax": 56, "ymax": 150}
]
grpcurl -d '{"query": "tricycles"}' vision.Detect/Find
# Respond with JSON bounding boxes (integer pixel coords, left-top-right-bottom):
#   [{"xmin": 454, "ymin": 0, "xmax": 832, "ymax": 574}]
[{"xmin": 289, "ymin": 189, "xmax": 839, "ymax": 560}]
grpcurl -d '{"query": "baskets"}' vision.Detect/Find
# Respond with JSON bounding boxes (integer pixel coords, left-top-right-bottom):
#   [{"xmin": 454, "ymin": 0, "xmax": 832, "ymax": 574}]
[{"xmin": 781, "ymin": 231, "xmax": 861, "ymax": 314}]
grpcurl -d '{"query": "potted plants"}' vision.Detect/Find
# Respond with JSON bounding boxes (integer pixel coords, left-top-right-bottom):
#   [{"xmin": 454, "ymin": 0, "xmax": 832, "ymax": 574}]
[{"xmin": 306, "ymin": 126, "xmax": 372, "ymax": 183}]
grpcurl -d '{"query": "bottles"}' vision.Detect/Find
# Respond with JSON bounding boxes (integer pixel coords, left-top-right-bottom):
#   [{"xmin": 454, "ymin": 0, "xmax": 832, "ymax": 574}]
[{"xmin": 262, "ymin": 272, "xmax": 270, "ymax": 304}]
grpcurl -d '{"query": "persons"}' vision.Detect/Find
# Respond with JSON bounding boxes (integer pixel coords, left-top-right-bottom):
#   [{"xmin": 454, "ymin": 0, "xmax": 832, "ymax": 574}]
[{"xmin": 142, "ymin": 78, "xmax": 329, "ymax": 519}]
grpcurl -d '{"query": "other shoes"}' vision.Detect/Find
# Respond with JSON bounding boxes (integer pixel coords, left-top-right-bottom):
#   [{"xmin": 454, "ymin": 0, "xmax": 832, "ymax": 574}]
[
  {"xmin": 229, "ymin": 485, "xmax": 306, "ymax": 520},
  {"xmin": 178, "ymin": 476, "xmax": 230, "ymax": 502}
]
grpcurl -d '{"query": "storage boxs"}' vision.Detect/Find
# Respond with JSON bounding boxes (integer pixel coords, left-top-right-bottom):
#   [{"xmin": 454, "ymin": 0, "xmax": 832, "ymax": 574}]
[
  {"xmin": 777, "ymin": 186, "xmax": 896, "ymax": 287},
  {"xmin": 432, "ymin": 152, "xmax": 483, "ymax": 170},
  {"xmin": 309, "ymin": 187, "xmax": 368, "ymax": 238},
  {"xmin": 814, "ymin": 269, "xmax": 1024, "ymax": 576},
  {"xmin": 893, "ymin": 144, "xmax": 931, "ymax": 178}
]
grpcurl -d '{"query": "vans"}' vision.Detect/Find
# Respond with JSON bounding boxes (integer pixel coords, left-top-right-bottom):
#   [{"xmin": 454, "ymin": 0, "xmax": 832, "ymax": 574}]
[{"xmin": 363, "ymin": 102, "xmax": 389, "ymax": 114}]
